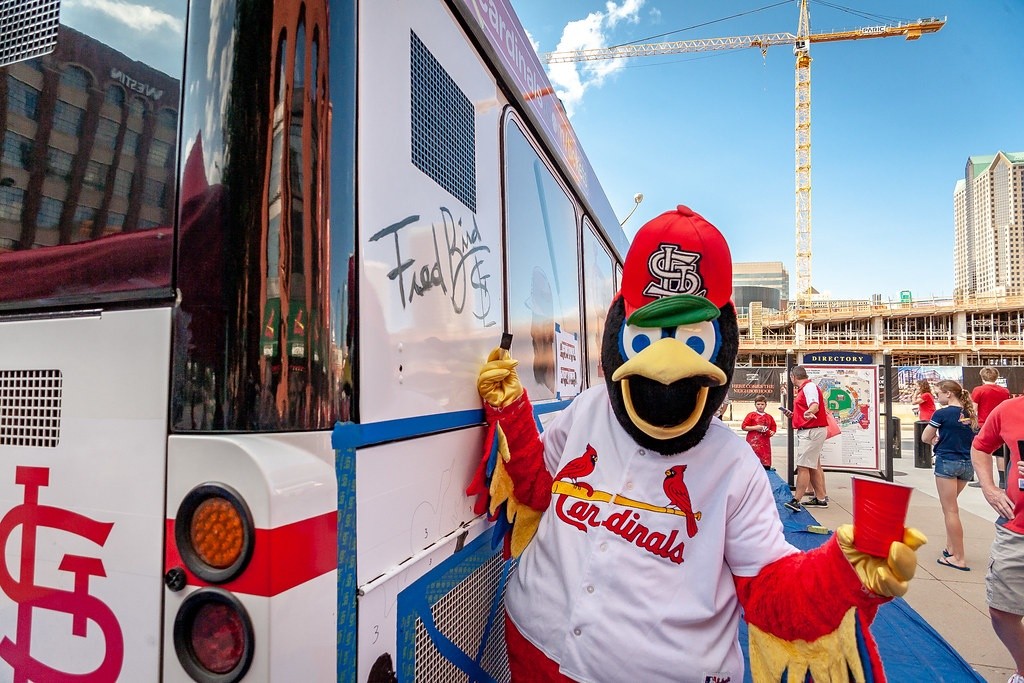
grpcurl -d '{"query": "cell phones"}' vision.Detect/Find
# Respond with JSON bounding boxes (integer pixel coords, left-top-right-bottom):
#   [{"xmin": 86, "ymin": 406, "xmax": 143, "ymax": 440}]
[{"xmin": 778, "ymin": 406, "xmax": 785, "ymax": 411}]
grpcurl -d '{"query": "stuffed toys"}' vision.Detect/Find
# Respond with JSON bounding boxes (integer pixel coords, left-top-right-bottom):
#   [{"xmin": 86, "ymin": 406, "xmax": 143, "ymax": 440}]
[{"xmin": 466, "ymin": 205, "xmax": 925, "ymax": 683}]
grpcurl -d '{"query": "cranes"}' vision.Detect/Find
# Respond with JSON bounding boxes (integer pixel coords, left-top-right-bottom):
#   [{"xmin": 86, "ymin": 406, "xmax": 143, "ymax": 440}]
[{"xmin": 537, "ymin": 0, "xmax": 949, "ymax": 314}]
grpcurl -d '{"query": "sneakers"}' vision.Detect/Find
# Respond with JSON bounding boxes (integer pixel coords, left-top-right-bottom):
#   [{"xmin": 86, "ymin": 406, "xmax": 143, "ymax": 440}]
[
  {"xmin": 784, "ymin": 497, "xmax": 801, "ymax": 512},
  {"xmin": 800, "ymin": 497, "xmax": 828, "ymax": 508}
]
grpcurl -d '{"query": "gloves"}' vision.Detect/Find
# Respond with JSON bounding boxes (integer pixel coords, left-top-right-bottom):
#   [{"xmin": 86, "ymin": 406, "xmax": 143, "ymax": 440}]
[
  {"xmin": 477, "ymin": 346, "xmax": 523, "ymax": 409},
  {"xmin": 836, "ymin": 523, "xmax": 926, "ymax": 598}
]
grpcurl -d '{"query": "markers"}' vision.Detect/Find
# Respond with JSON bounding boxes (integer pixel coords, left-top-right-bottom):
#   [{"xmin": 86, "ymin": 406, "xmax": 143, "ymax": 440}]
[{"xmin": 494, "ymin": 332, "xmax": 514, "ymax": 388}]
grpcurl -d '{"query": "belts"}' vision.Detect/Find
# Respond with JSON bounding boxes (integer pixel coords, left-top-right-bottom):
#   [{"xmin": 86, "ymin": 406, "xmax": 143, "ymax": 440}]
[{"xmin": 798, "ymin": 426, "xmax": 825, "ymax": 430}]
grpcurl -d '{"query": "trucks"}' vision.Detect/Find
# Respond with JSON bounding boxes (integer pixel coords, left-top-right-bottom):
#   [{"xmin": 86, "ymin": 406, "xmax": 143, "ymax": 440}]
[{"xmin": 1, "ymin": 2, "xmax": 639, "ymax": 683}]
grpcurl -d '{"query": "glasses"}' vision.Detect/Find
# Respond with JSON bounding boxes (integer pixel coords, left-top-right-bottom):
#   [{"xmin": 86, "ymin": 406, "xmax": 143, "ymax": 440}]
[{"xmin": 935, "ymin": 391, "xmax": 944, "ymax": 396}]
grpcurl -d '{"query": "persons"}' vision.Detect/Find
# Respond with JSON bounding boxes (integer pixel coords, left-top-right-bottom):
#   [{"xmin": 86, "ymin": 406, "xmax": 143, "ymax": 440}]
[
  {"xmin": 923, "ymin": 380, "xmax": 975, "ymax": 571},
  {"xmin": 968, "ymin": 365, "xmax": 1012, "ymax": 488},
  {"xmin": 971, "ymin": 395, "xmax": 1024, "ymax": 683},
  {"xmin": 912, "ymin": 378, "xmax": 936, "ymax": 421},
  {"xmin": 741, "ymin": 395, "xmax": 777, "ymax": 470},
  {"xmin": 779, "ymin": 381, "xmax": 788, "ymax": 408},
  {"xmin": 783, "ymin": 366, "xmax": 829, "ymax": 512},
  {"xmin": 524, "ymin": 266, "xmax": 555, "ymax": 393}
]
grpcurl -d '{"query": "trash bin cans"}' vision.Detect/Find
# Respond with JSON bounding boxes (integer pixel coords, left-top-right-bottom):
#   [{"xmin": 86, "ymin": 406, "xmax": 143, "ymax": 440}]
[
  {"xmin": 891, "ymin": 416, "xmax": 902, "ymax": 459},
  {"xmin": 913, "ymin": 420, "xmax": 932, "ymax": 469}
]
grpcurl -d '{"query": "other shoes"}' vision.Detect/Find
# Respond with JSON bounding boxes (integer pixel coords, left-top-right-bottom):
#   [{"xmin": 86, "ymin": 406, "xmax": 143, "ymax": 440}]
[
  {"xmin": 968, "ymin": 481, "xmax": 980, "ymax": 488},
  {"xmin": 1008, "ymin": 673, "xmax": 1023, "ymax": 683},
  {"xmin": 808, "ymin": 496, "xmax": 829, "ymax": 503}
]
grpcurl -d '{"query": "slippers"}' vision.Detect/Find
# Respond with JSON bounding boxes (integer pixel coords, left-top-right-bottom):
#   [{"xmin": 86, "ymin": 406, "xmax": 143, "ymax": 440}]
[
  {"xmin": 943, "ymin": 548, "xmax": 952, "ymax": 557},
  {"xmin": 937, "ymin": 556, "xmax": 970, "ymax": 571}
]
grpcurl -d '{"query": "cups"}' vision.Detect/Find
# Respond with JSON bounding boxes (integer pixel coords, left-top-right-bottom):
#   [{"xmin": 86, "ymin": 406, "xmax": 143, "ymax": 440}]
[{"xmin": 850, "ymin": 476, "xmax": 914, "ymax": 558}]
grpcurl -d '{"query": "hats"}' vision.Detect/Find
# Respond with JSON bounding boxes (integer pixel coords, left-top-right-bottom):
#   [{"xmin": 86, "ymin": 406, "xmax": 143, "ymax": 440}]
[{"xmin": 621, "ymin": 204, "xmax": 733, "ymax": 328}]
